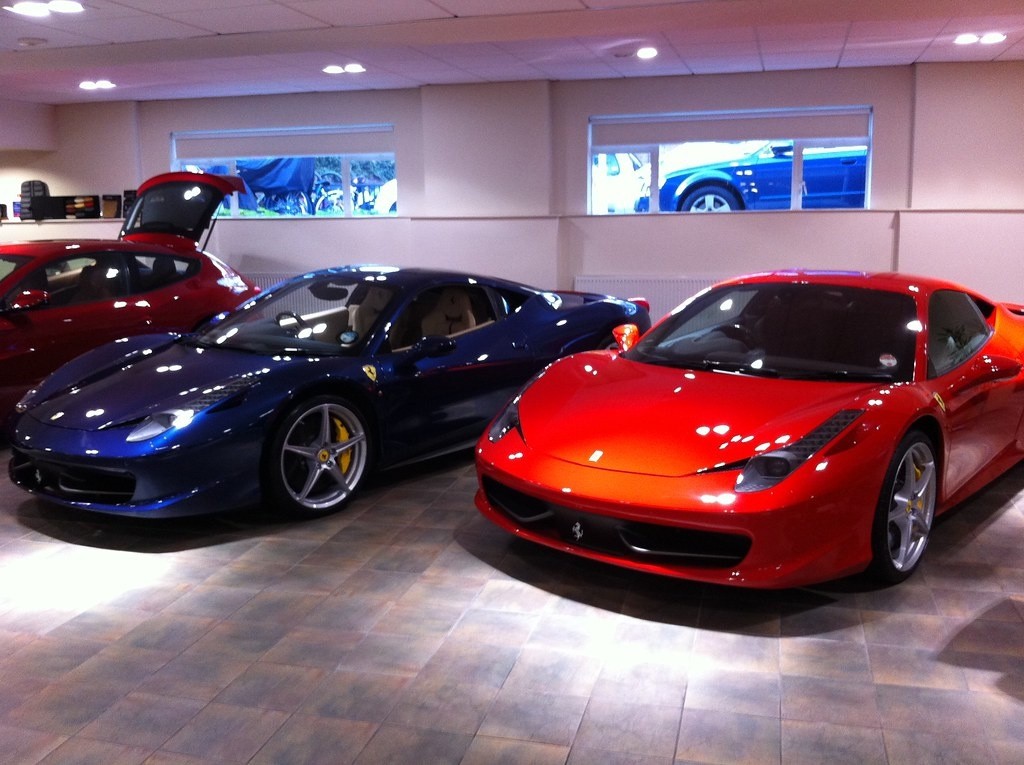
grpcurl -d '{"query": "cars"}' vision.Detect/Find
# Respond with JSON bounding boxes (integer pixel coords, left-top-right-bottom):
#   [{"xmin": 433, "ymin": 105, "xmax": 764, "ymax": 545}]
[
  {"xmin": 0, "ymin": 170, "xmax": 265, "ymax": 439},
  {"xmin": 641, "ymin": 135, "xmax": 867, "ymax": 210},
  {"xmin": 7, "ymin": 265, "xmax": 651, "ymax": 533},
  {"xmin": 471, "ymin": 264, "xmax": 1023, "ymax": 590},
  {"xmin": 375, "ymin": 151, "xmax": 652, "ymax": 213}
]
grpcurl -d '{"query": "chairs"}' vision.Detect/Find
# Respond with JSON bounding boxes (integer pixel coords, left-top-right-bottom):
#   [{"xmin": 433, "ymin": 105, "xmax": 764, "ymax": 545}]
[
  {"xmin": 69, "ymin": 265, "xmax": 113, "ymax": 303},
  {"xmin": 138, "ymin": 256, "xmax": 183, "ymax": 292},
  {"xmin": 353, "ymin": 285, "xmax": 412, "ymax": 349},
  {"xmin": 419, "ymin": 289, "xmax": 477, "ymax": 336}
]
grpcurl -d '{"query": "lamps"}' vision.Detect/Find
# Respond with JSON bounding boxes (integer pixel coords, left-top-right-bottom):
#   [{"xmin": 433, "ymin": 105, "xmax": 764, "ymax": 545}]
[
  {"xmin": 980, "ymin": 33, "xmax": 1007, "ymax": 44},
  {"xmin": 79, "ymin": 81, "xmax": 96, "ymax": 90},
  {"xmin": 95, "ymin": 81, "xmax": 117, "ymax": 89},
  {"xmin": 47, "ymin": 0, "xmax": 85, "ymax": 13},
  {"xmin": 322, "ymin": 65, "xmax": 345, "ymax": 74},
  {"xmin": 344, "ymin": 64, "xmax": 367, "ymax": 73},
  {"xmin": 954, "ymin": 33, "xmax": 980, "ymax": 45},
  {"xmin": 2, "ymin": 0, "xmax": 50, "ymax": 17},
  {"xmin": 636, "ymin": 47, "xmax": 657, "ymax": 59}
]
